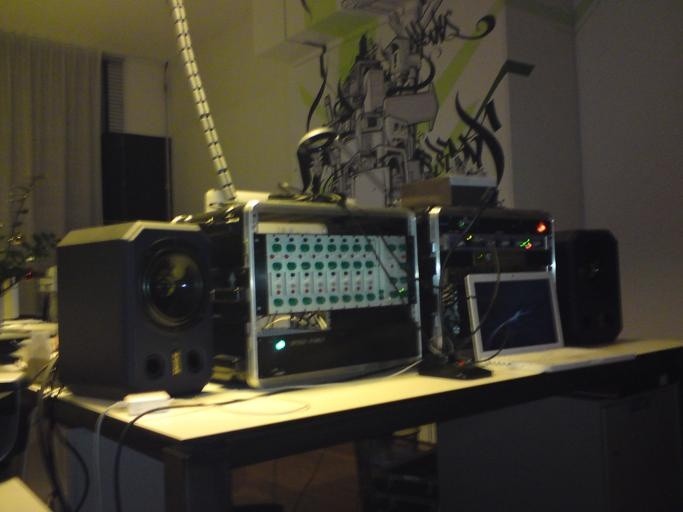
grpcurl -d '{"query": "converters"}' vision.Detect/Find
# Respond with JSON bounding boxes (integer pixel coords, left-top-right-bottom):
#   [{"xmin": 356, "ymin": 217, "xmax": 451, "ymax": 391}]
[{"xmin": 123, "ymin": 391, "xmax": 172, "ymax": 416}]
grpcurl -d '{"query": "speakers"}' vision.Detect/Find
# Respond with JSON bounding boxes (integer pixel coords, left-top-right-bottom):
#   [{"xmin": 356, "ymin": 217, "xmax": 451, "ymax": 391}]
[
  {"xmin": 55, "ymin": 220, "xmax": 214, "ymax": 401},
  {"xmin": 555, "ymin": 229, "xmax": 623, "ymax": 348}
]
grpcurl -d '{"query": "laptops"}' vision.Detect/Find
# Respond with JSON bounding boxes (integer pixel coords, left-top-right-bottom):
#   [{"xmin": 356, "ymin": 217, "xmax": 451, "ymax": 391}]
[{"xmin": 464, "ymin": 271, "xmax": 636, "ymax": 374}]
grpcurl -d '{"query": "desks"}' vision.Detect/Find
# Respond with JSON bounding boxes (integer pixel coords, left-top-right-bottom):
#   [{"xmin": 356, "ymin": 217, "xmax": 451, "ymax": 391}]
[{"xmin": 16, "ymin": 340, "xmax": 683, "ymax": 512}]
